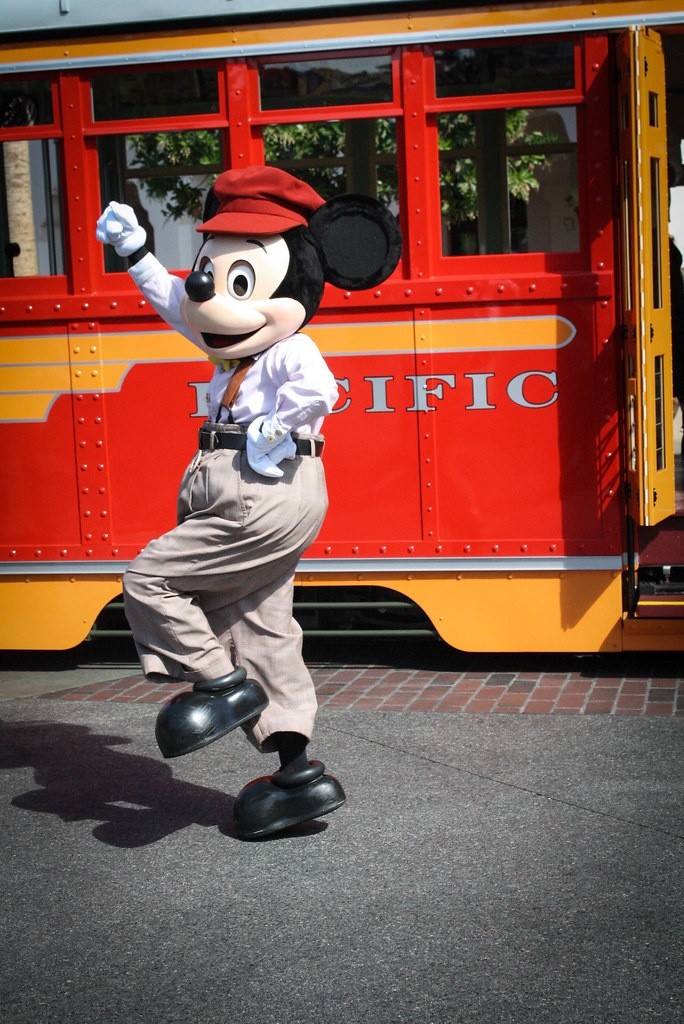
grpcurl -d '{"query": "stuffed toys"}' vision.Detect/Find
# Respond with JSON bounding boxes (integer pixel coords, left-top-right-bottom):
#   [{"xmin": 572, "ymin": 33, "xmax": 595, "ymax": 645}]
[{"xmin": 94, "ymin": 168, "xmax": 403, "ymax": 839}]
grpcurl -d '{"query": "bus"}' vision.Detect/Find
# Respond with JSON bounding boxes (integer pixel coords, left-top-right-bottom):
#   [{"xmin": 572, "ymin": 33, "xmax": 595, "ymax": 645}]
[{"xmin": 0, "ymin": 1, "xmax": 684, "ymax": 659}]
